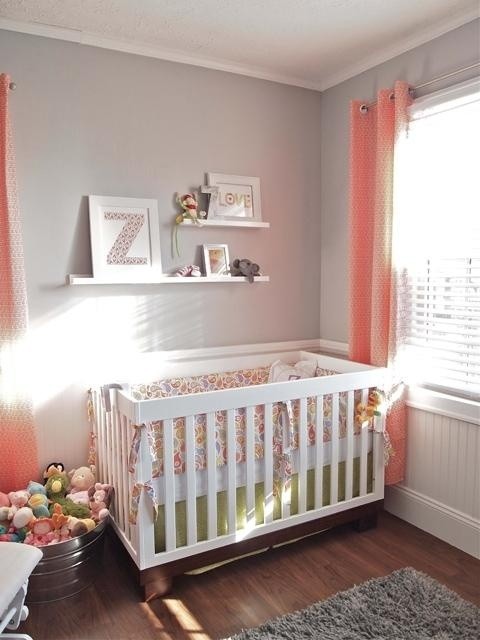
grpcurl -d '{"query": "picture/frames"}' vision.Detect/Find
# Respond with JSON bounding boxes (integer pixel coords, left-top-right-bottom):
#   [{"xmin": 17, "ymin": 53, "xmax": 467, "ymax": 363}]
[
  {"xmin": 202, "ymin": 244, "xmax": 231, "ymax": 277},
  {"xmin": 206, "ymin": 172, "xmax": 261, "ymax": 221},
  {"xmin": 89, "ymin": 196, "xmax": 162, "ymax": 279}
]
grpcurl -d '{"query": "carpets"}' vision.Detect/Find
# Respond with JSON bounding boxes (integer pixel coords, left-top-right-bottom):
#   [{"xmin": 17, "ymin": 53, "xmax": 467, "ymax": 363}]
[{"xmin": 219, "ymin": 566, "xmax": 480, "ymax": 640}]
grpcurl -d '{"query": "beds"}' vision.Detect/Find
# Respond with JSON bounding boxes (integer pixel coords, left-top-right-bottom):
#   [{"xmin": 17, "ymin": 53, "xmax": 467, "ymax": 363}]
[{"xmin": 94, "ymin": 350, "xmax": 387, "ymax": 603}]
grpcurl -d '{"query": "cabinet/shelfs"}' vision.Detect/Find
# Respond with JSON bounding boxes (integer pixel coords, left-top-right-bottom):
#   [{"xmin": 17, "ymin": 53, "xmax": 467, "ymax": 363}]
[{"xmin": 69, "ymin": 218, "xmax": 270, "ymax": 285}]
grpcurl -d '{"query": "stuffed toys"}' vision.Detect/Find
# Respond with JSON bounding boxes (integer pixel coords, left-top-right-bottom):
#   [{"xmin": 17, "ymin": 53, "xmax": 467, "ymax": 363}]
[
  {"xmin": 354, "ymin": 390, "xmax": 380, "ymax": 429},
  {"xmin": 231, "ymin": 258, "xmax": 260, "ymax": 283},
  {"xmin": 174, "ymin": 191, "xmax": 203, "ymax": 256},
  {"xmin": 175, "ymin": 264, "xmax": 202, "ymax": 277},
  {"xmin": 0, "ymin": 462, "xmax": 114, "ymax": 548}
]
